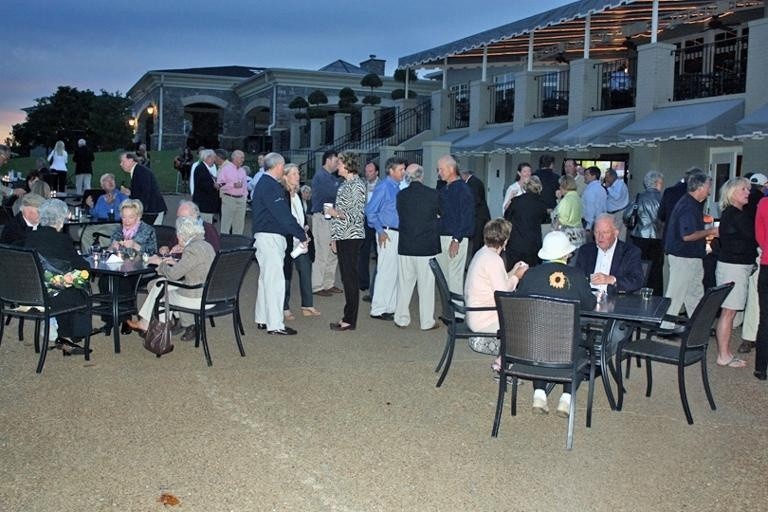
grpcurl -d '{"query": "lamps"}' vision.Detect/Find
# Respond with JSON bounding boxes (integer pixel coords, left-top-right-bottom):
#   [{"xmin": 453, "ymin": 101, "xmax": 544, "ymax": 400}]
[
  {"xmin": 128, "ymin": 115, "xmax": 134, "ymax": 126},
  {"xmin": 146, "ymin": 102, "xmax": 153, "ymax": 114}
]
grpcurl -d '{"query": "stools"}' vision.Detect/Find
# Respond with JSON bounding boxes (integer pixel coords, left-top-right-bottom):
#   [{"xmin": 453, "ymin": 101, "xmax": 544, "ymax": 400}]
[{"xmin": 175, "ymin": 171, "xmax": 190, "ymax": 194}]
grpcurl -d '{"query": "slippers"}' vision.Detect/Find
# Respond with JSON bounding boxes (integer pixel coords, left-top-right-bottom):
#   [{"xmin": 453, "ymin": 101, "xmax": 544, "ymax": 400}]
[{"xmin": 715, "ymin": 352, "xmax": 748, "ymax": 368}]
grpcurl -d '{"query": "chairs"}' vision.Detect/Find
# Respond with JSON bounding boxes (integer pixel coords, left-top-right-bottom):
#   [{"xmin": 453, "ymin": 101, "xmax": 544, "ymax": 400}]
[
  {"xmin": 428, "ymin": 257, "xmax": 498, "ymax": 388},
  {"xmin": 0, "ymin": 167, "xmax": 121, "ymax": 342},
  {"xmin": 120, "ymin": 212, "xmax": 159, "ymax": 225},
  {"xmin": 152, "ymin": 225, "xmax": 174, "ymax": 294},
  {"xmin": 626, "ymin": 232, "xmax": 665, "ymax": 378},
  {"xmin": 164, "ymin": 232, "xmax": 256, "ymax": 336},
  {"xmin": 152, "ymin": 246, "xmax": 257, "ymax": 366},
  {"xmin": 616, "ymin": 281, "xmax": 736, "ymax": 424},
  {"xmin": 490, "ymin": 289, "xmax": 597, "ymax": 451},
  {"xmin": 0, "ymin": 244, "xmax": 92, "ymax": 373}
]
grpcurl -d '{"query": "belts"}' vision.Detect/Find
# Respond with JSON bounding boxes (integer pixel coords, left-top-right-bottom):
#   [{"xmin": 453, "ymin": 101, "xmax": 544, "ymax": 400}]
[
  {"xmin": 381, "ymin": 226, "xmax": 400, "ymax": 231},
  {"xmin": 607, "ymin": 203, "xmax": 632, "ymax": 214},
  {"xmin": 223, "ymin": 193, "xmax": 244, "ymax": 198}
]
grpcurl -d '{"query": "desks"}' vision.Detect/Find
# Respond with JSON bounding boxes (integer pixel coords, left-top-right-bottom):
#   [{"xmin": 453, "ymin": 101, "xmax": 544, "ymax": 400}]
[
  {"xmin": 580, "ymin": 286, "xmax": 672, "ymax": 412},
  {"xmin": 82, "ymin": 248, "xmax": 170, "ymax": 354}
]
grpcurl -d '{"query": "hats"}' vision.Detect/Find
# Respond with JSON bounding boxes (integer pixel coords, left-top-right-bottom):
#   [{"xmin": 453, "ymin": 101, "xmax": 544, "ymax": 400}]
[
  {"xmin": 748, "ymin": 173, "xmax": 768, "ymax": 186},
  {"xmin": 537, "ymin": 231, "xmax": 577, "ymax": 260}
]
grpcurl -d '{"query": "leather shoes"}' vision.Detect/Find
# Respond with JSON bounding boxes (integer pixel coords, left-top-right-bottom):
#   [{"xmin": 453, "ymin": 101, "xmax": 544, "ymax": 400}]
[
  {"xmin": 491, "ymin": 361, "xmax": 524, "ymax": 386},
  {"xmin": 55, "ymin": 336, "xmax": 93, "ymax": 356},
  {"xmin": 753, "ymin": 370, "xmax": 767, "ymax": 379},
  {"xmin": 100, "ymin": 319, "xmax": 196, "ymax": 341},
  {"xmin": 259, "ymin": 286, "xmax": 464, "ymax": 335},
  {"xmin": 739, "ymin": 340, "xmax": 752, "ymax": 353}
]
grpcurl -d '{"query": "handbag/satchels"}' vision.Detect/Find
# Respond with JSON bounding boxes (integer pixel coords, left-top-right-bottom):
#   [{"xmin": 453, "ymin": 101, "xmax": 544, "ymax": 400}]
[
  {"xmin": 144, "ymin": 315, "xmax": 173, "ymax": 355},
  {"xmin": 626, "ymin": 210, "xmax": 638, "ymax": 231}
]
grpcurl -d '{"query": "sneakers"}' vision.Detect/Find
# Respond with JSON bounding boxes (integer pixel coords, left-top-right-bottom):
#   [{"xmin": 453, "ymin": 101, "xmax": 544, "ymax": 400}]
[
  {"xmin": 556, "ymin": 400, "xmax": 570, "ymax": 418},
  {"xmin": 531, "ymin": 398, "xmax": 549, "ymax": 415}
]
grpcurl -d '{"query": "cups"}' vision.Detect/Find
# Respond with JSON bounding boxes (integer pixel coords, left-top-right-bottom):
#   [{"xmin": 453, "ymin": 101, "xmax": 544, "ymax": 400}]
[
  {"xmin": 589, "ymin": 273, "xmax": 598, "ymax": 286},
  {"xmin": 642, "ymin": 288, "xmax": 654, "ymax": 300},
  {"xmin": 713, "ymin": 217, "xmax": 721, "ymax": 239},
  {"xmin": 323, "ymin": 203, "xmax": 333, "ymax": 221},
  {"xmin": 90, "ymin": 244, "xmax": 150, "ymax": 268}
]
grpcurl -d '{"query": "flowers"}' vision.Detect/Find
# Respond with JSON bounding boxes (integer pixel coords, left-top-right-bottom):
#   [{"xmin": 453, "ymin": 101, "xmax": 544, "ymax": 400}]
[{"xmin": 549, "ymin": 271, "xmax": 570, "ymax": 291}]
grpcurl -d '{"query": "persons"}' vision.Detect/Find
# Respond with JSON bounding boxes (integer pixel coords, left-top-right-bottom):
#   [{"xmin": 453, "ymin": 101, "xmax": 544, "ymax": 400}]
[
  {"xmin": 213, "ymin": 148, "xmax": 251, "ymax": 234},
  {"xmin": 0, "ymin": 137, "xmax": 218, "ymax": 355},
  {"xmin": 246, "ymin": 150, "xmax": 488, "ymax": 334},
  {"xmin": 626, "ymin": 167, "xmax": 768, "ymax": 381},
  {"xmin": 505, "ymin": 154, "xmax": 630, "ymax": 266},
  {"xmin": 464, "ymin": 217, "xmax": 531, "ymax": 386},
  {"xmin": 517, "ymin": 230, "xmax": 598, "ymax": 418}
]
